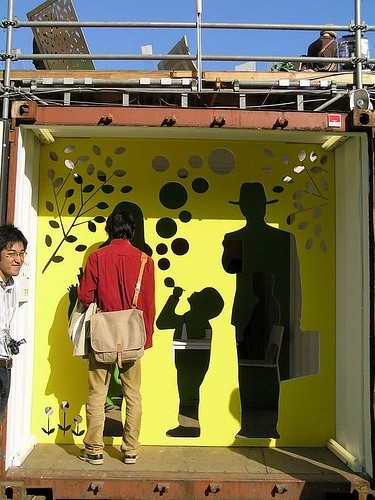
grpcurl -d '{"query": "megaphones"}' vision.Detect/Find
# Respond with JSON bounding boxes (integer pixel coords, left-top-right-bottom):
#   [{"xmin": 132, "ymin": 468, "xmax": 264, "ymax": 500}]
[{"xmin": 349, "ymin": 89, "xmax": 373, "ymax": 111}]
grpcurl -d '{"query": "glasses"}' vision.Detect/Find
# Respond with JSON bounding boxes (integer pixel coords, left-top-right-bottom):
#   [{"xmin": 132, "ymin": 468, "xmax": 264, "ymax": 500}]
[{"xmin": 1, "ymin": 252, "xmax": 27, "ymax": 258}]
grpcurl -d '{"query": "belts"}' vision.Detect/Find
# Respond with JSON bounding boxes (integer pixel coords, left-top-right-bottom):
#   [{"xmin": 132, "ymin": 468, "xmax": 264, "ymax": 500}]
[{"xmin": 0, "ymin": 359, "xmax": 13, "ymax": 369}]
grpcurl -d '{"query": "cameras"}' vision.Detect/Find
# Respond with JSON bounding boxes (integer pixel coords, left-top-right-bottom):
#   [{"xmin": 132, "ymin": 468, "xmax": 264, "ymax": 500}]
[{"xmin": 7, "ymin": 338, "xmax": 26, "ymax": 355}]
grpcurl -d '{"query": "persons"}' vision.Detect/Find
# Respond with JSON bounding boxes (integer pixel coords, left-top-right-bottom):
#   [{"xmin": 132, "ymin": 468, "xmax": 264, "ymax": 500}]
[
  {"xmin": 1, "ymin": 225, "xmax": 27, "ymax": 422},
  {"xmin": 308, "ymin": 23, "xmax": 340, "ymax": 72},
  {"xmin": 77, "ymin": 210, "xmax": 155, "ymax": 465}
]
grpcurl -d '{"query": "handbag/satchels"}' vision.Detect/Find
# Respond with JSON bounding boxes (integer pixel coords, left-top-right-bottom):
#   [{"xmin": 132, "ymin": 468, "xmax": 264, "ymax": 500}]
[{"xmin": 90, "ymin": 252, "xmax": 147, "ymax": 368}]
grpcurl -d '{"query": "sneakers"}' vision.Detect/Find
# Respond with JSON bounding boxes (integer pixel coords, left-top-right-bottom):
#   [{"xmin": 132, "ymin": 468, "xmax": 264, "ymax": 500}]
[
  {"xmin": 124, "ymin": 454, "xmax": 137, "ymax": 464},
  {"xmin": 78, "ymin": 451, "xmax": 103, "ymax": 464}
]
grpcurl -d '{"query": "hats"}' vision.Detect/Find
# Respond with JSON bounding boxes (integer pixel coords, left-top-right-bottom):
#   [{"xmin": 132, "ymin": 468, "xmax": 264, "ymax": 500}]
[{"xmin": 320, "ymin": 23, "xmax": 337, "ymax": 39}]
